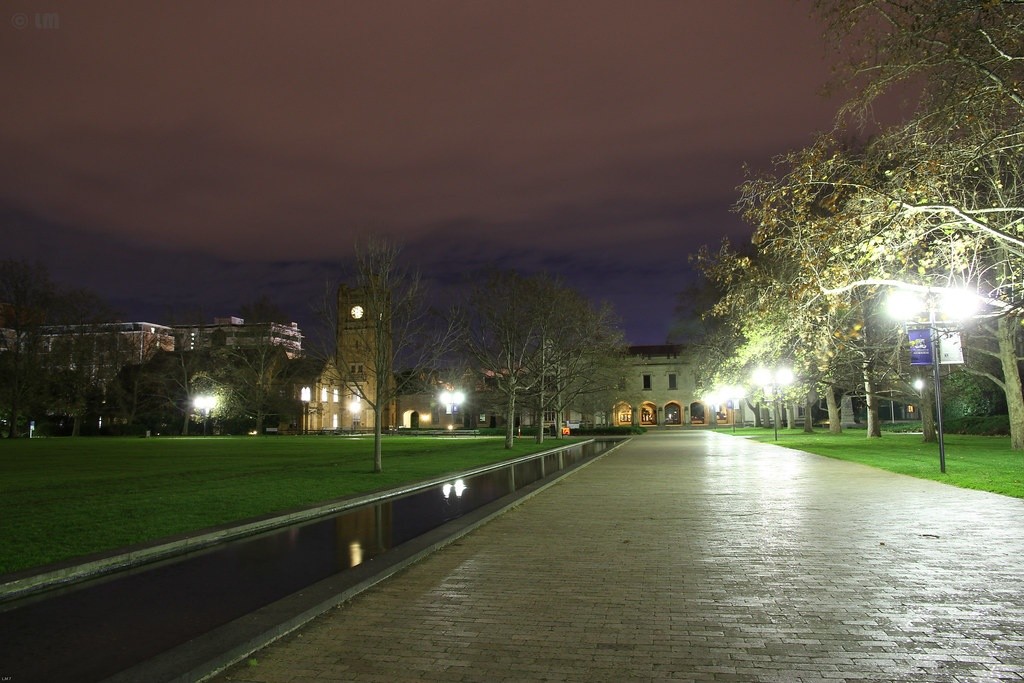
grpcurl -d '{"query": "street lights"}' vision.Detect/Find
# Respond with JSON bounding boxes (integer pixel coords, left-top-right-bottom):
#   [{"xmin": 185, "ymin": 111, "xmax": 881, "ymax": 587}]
[
  {"xmin": 720, "ymin": 387, "xmax": 744, "ymax": 433},
  {"xmin": 757, "ymin": 367, "xmax": 792, "ymax": 441},
  {"xmin": 442, "ymin": 392, "xmax": 462, "ymax": 438},
  {"xmin": 890, "ymin": 291, "xmax": 975, "ymax": 473},
  {"xmin": 196, "ymin": 397, "xmax": 214, "ymax": 438}
]
